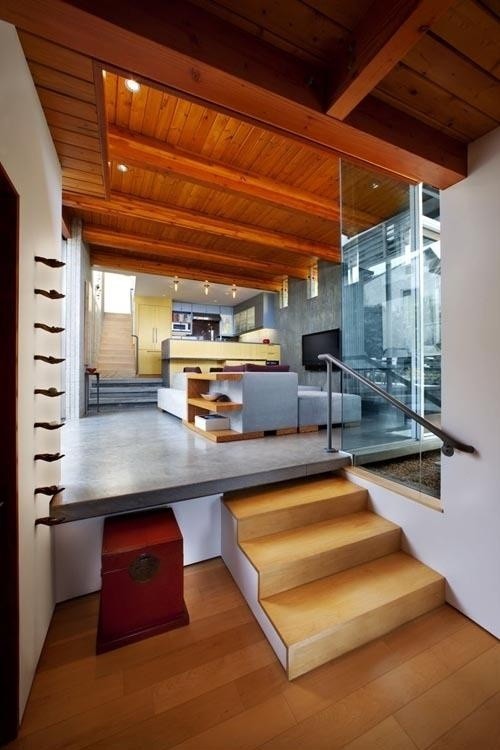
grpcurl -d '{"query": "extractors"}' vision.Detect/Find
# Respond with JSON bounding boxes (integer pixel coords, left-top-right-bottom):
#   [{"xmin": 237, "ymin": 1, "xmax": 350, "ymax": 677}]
[{"xmin": 193, "ymin": 313, "xmax": 222, "ymax": 322}]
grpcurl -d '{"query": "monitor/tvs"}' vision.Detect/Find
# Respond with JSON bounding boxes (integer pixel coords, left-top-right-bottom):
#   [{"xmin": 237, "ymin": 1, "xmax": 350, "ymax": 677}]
[{"xmin": 302, "ymin": 328, "xmax": 341, "ymax": 371}]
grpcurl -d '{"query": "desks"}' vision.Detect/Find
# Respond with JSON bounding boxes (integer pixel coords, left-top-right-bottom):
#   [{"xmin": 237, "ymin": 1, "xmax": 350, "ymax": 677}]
[{"xmin": 85, "ymin": 372, "xmax": 99, "ymax": 414}]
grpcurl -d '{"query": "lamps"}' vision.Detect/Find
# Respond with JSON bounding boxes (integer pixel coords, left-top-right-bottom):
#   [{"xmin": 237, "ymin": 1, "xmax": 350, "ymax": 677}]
[
  {"xmin": 232, "ymin": 284, "xmax": 237, "ymax": 298},
  {"xmin": 172, "ymin": 275, "xmax": 179, "ymax": 293},
  {"xmin": 203, "ymin": 280, "xmax": 210, "ymax": 296}
]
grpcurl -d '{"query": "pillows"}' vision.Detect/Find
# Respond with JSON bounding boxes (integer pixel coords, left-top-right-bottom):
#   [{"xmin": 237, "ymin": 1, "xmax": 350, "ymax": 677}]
[
  {"xmin": 183, "ymin": 367, "xmax": 202, "ymax": 373},
  {"xmin": 223, "ymin": 364, "xmax": 245, "ymax": 372},
  {"xmin": 246, "ymin": 364, "xmax": 290, "ymax": 371}
]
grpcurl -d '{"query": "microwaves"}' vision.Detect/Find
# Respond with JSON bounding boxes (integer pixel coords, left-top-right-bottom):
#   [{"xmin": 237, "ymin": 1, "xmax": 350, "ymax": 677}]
[{"xmin": 172, "ymin": 322, "xmax": 190, "ymax": 332}]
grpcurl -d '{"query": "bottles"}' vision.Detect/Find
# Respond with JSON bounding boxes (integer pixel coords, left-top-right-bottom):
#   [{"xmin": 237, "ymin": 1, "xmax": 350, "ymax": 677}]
[{"xmin": 207, "ymin": 324, "xmax": 214, "ymax": 341}]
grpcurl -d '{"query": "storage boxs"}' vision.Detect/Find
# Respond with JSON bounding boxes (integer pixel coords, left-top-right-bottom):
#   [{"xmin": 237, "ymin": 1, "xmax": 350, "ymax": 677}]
[
  {"xmin": 193, "ymin": 413, "xmax": 231, "ymax": 432},
  {"xmin": 95, "ymin": 507, "xmax": 190, "ymax": 655}
]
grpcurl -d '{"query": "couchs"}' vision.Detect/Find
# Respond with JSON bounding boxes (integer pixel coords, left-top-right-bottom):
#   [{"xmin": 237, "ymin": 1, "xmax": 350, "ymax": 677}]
[{"xmin": 157, "ymin": 362, "xmax": 362, "ymax": 444}]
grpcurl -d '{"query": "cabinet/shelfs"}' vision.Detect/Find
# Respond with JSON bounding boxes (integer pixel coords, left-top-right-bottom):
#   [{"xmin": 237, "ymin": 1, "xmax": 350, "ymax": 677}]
[
  {"xmin": 219, "ymin": 305, "xmax": 234, "ymax": 338},
  {"xmin": 234, "ymin": 292, "xmax": 275, "ymax": 337},
  {"xmin": 172, "ymin": 302, "xmax": 192, "ymax": 314},
  {"xmin": 137, "ymin": 303, "xmax": 171, "ymax": 375},
  {"xmin": 192, "ymin": 304, "xmax": 219, "ymax": 314}
]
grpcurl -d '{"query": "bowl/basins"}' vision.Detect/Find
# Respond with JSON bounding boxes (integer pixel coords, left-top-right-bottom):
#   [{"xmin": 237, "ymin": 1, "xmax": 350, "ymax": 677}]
[{"xmin": 201, "ymin": 392, "xmax": 222, "ymax": 403}]
